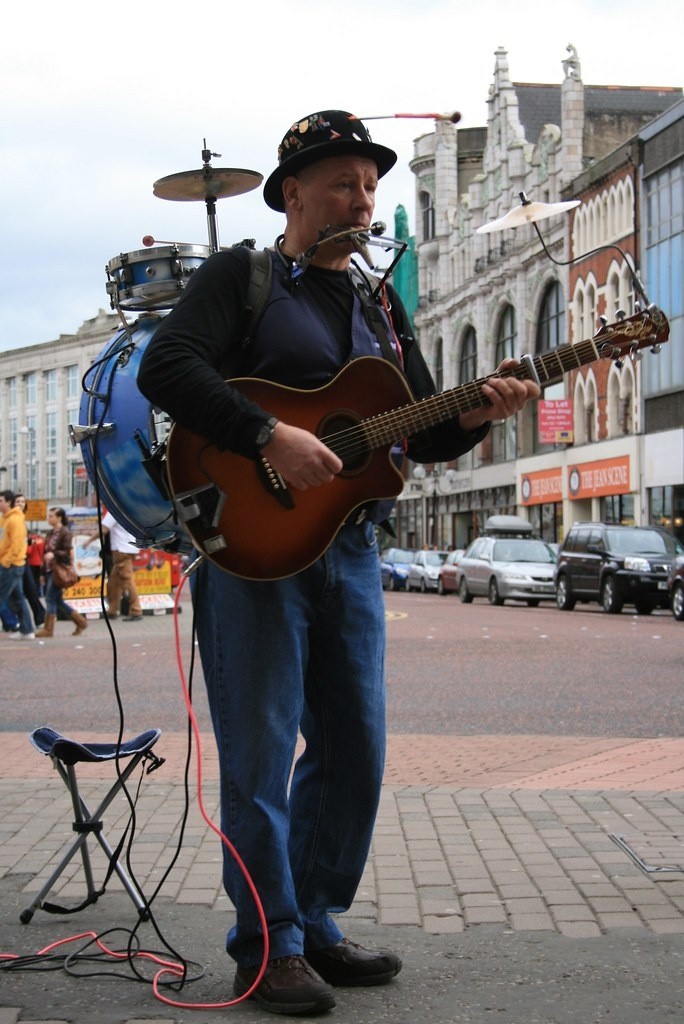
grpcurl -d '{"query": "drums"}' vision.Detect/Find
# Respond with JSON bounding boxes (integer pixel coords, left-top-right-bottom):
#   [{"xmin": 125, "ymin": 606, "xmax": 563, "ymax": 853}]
[
  {"xmin": 74, "ymin": 313, "xmax": 214, "ymax": 556},
  {"xmin": 104, "ymin": 243, "xmax": 211, "ymax": 312}
]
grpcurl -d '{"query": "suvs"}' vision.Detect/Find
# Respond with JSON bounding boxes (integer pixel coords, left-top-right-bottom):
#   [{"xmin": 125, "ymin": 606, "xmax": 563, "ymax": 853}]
[
  {"xmin": 553, "ymin": 521, "xmax": 684, "ymax": 621},
  {"xmin": 456, "ymin": 515, "xmax": 557, "ymax": 607}
]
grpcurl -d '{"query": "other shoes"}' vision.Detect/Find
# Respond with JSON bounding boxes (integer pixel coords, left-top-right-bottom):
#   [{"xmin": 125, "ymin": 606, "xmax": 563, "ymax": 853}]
[
  {"xmin": 99, "ymin": 611, "xmax": 119, "ymax": 620},
  {"xmin": 121, "ymin": 613, "xmax": 141, "ymax": 621}
]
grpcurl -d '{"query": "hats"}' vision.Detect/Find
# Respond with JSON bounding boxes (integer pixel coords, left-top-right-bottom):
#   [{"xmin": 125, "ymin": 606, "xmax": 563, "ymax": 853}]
[{"xmin": 264, "ymin": 110, "xmax": 399, "ymax": 212}]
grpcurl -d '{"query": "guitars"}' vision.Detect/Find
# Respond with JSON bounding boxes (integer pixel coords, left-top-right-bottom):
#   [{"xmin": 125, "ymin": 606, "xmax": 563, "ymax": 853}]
[{"xmin": 161, "ymin": 300, "xmax": 672, "ymax": 582}]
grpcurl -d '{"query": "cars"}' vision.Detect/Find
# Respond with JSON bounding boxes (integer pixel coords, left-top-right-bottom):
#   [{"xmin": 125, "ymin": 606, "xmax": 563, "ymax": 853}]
[{"xmin": 379, "ymin": 547, "xmax": 466, "ymax": 595}]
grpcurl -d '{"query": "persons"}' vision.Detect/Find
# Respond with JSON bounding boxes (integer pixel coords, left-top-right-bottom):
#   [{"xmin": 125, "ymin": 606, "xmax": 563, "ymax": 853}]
[
  {"xmin": 422, "ymin": 543, "xmax": 454, "ymax": 551},
  {"xmin": 136, "ymin": 111, "xmax": 540, "ymax": 1014},
  {"xmin": 0, "ymin": 490, "xmax": 89, "ymax": 640},
  {"xmin": 83, "ymin": 511, "xmax": 142, "ymax": 622}
]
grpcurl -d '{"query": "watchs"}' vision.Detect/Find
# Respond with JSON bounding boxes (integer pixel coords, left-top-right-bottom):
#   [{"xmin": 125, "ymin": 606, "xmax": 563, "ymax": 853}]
[{"xmin": 256, "ymin": 415, "xmax": 279, "ymax": 450}]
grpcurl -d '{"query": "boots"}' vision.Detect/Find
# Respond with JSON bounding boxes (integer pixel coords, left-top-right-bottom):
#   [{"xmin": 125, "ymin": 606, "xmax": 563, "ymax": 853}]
[
  {"xmin": 34, "ymin": 611, "xmax": 57, "ymax": 638},
  {"xmin": 68, "ymin": 609, "xmax": 88, "ymax": 636}
]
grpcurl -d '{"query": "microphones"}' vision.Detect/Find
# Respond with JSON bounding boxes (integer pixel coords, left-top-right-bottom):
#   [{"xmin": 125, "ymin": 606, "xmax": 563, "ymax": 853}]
[{"xmin": 371, "ymin": 221, "xmax": 387, "ymax": 236}]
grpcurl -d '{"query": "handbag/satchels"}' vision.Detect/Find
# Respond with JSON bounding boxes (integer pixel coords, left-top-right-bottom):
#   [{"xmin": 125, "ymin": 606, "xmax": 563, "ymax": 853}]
[{"xmin": 51, "ymin": 562, "xmax": 78, "ymax": 588}]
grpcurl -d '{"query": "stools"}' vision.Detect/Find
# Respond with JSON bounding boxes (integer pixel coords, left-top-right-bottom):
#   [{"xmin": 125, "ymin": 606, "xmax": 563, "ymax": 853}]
[{"xmin": 20, "ymin": 724, "xmax": 162, "ymax": 923}]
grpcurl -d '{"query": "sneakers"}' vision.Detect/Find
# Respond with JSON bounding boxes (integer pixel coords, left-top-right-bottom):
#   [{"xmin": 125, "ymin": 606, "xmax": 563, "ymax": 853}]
[
  {"xmin": 9, "ymin": 631, "xmax": 36, "ymax": 641},
  {"xmin": 302, "ymin": 932, "xmax": 403, "ymax": 988},
  {"xmin": 234, "ymin": 949, "xmax": 336, "ymax": 1017}
]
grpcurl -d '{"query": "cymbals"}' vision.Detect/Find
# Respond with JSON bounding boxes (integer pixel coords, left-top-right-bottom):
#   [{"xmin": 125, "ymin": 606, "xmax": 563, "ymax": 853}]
[
  {"xmin": 474, "ymin": 198, "xmax": 583, "ymax": 235},
  {"xmin": 152, "ymin": 167, "xmax": 265, "ymax": 202}
]
